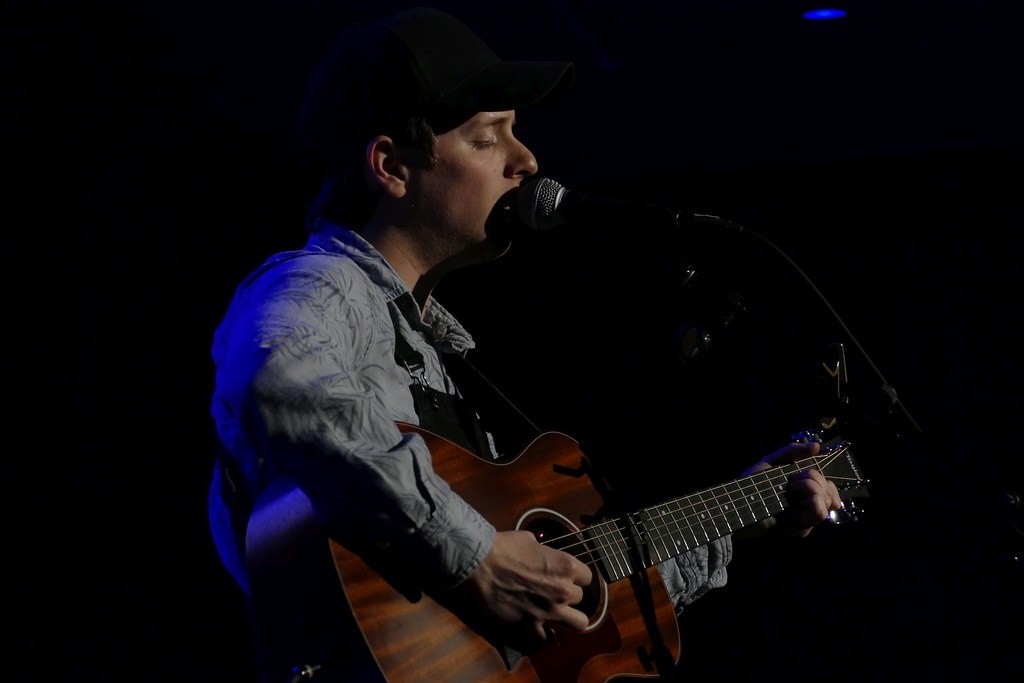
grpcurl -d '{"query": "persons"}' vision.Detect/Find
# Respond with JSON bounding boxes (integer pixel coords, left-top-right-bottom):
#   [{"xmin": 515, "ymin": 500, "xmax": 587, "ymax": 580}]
[{"xmin": 206, "ymin": 8, "xmax": 843, "ymax": 682}]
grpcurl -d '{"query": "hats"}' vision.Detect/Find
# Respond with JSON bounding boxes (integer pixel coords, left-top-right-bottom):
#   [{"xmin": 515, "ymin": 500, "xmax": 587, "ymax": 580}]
[{"xmin": 297, "ymin": 8, "xmax": 571, "ymax": 171}]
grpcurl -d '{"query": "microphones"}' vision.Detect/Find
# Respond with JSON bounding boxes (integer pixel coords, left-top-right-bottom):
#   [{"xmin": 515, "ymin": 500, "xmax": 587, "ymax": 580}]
[{"xmin": 519, "ymin": 177, "xmax": 744, "ymax": 234}]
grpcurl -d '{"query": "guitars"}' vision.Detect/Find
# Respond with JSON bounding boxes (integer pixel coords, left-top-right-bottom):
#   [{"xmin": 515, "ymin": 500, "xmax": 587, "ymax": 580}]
[{"xmin": 323, "ymin": 412, "xmax": 876, "ymax": 683}]
